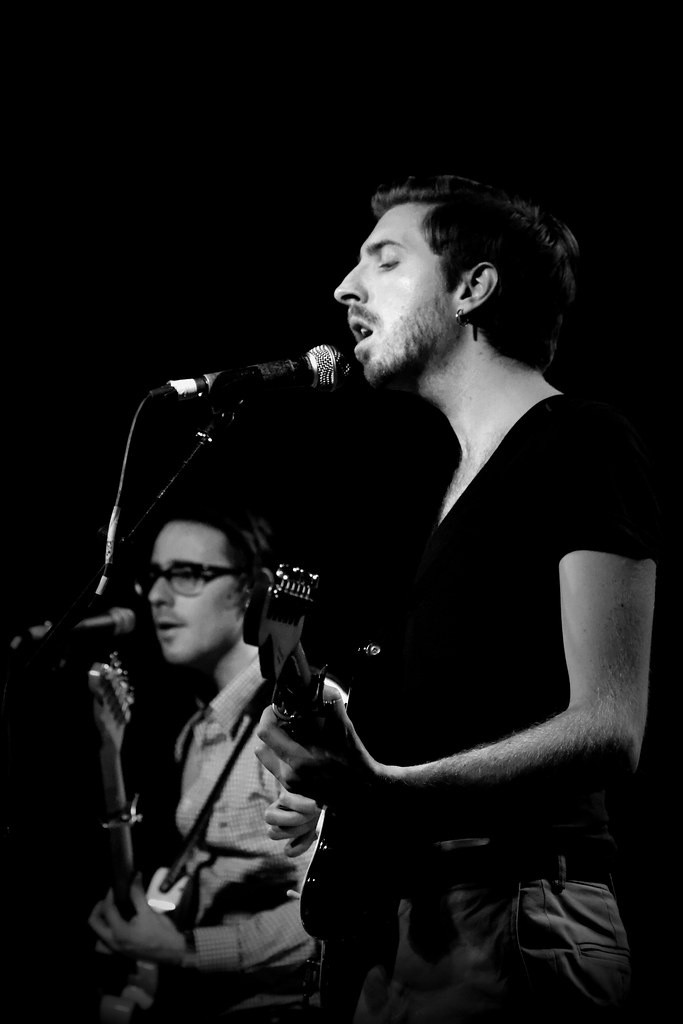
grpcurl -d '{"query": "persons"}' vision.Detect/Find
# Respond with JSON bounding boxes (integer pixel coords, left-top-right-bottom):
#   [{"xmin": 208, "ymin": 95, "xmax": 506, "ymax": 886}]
[
  {"xmin": 255, "ymin": 174, "xmax": 662, "ymax": 1024},
  {"xmin": 88, "ymin": 497, "xmax": 350, "ymax": 1024}
]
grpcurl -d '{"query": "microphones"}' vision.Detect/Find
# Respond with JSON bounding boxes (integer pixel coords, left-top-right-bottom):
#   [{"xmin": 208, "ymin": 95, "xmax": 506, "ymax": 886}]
[
  {"xmin": 28, "ymin": 607, "xmax": 136, "ymax": 642},
  {"xmin": 144, "ymin": 344, "xmax": 351, "ymax": 401}
]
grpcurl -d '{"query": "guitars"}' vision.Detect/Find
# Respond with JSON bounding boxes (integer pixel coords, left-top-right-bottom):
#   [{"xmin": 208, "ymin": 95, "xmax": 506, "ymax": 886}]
[
  {"xmin": 86, "ymin": 650, "xmax": 200, "ymax": 1024},
  {"xmin": 243, "ymin": 561, "xmax": 399, "ymax": 1024}
]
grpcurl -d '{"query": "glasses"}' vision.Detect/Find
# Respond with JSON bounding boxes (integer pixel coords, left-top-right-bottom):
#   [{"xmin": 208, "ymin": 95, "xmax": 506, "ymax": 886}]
[{"xmin": 131, "ymin": 559, "xmax": 243, "ymax": 598}]
